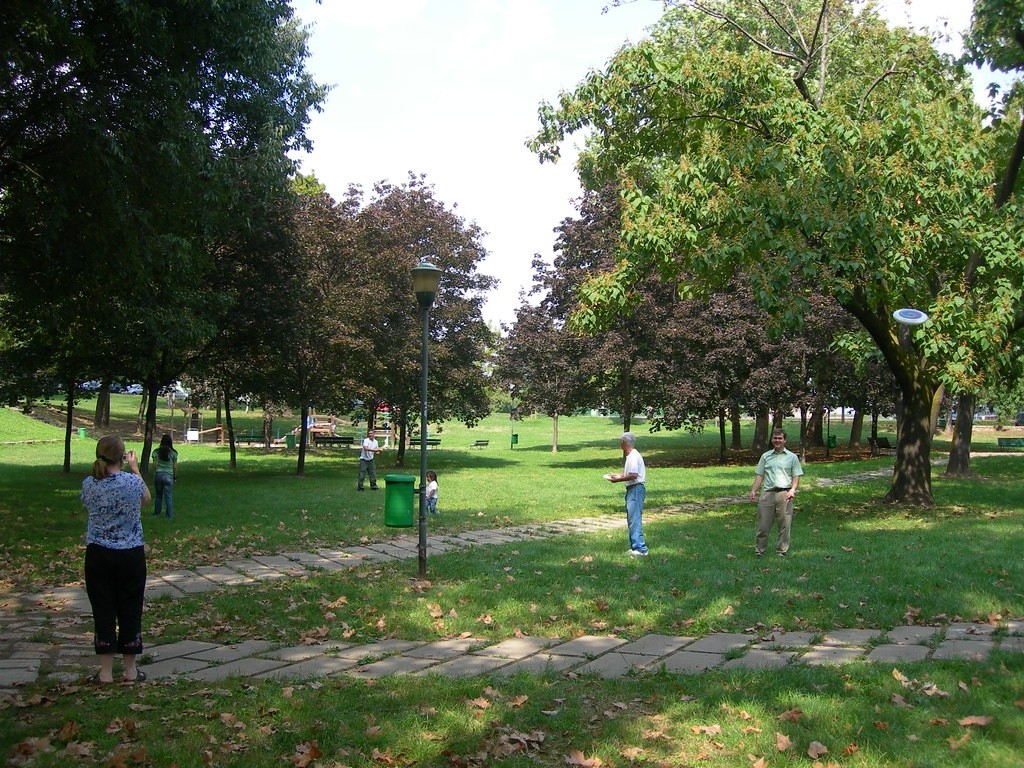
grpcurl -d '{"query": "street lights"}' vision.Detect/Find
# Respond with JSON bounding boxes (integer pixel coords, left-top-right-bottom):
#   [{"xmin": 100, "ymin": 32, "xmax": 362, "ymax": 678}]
[{"xmin": 411, "ymin": 260, "xmax": 444, "ymax": 586}]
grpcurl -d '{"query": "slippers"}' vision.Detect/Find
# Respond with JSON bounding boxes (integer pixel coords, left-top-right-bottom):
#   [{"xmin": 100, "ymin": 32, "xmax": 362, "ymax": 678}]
[
  {"xmin": 124, "ymin": 669, "xmax": 147, "ymax": 682},
  {"xmin": 87, "ymin": 672, "xmax": 112, "ymax": 685}
]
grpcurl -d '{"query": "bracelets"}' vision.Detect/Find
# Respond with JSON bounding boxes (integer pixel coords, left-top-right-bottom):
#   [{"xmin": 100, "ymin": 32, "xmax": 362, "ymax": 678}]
[
  {"xmin": 790, "ymin": 489, "xmax": 796, "ymax": 493},
  {"xmin": 174, "ymin": 476, "xmax": 177, "ymax": 479}
]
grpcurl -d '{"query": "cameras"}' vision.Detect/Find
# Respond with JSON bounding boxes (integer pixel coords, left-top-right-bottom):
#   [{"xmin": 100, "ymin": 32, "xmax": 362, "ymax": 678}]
[{"xmin": 123, "ymin": 455, "xmax": 128, "ymax": 460}]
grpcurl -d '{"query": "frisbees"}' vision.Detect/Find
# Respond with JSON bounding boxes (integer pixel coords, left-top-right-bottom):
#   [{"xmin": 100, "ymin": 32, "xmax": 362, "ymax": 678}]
[{"xmin": 603, "ymin": 473, "xmax": 619, "ymax": 481}]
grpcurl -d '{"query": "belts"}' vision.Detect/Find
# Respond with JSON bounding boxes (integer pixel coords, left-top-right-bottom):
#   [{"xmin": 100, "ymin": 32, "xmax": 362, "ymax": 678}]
[{"xmin": 766, "ymin": 487, "xmax": 791, "ymax": 492}]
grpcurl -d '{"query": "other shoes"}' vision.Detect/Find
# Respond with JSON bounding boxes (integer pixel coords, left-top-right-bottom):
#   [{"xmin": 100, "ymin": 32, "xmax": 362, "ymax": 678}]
[{"xmin": 625, "ymin": 549, "xmax": 648, "ymax": 556}]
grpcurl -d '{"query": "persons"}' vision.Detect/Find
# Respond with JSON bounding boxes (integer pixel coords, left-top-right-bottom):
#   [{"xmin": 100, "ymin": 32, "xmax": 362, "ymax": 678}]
[
  {"xmin": 80, "ymin": 435, "xmax": 153, "ymax": 685},
  {"xmin": 749, "ymin": 428, "xmax": 804, "ymax": 557},
  {"xmin": 357, "ymin": 429, "xmax": 383, "ymax": 491},
  {"xmin": 426, "ymin": 470, "xmax": 440, "ymax": 516},
  {"xmin": 151, "ymin": 434, "xmax": 178, "ymax": 517},
  {"xmin": 608, "ymin": 432, "xmax": 649, "ymax": 556}
]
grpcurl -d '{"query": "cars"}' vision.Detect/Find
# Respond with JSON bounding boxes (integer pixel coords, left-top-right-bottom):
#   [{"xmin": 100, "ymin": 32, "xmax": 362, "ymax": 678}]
[{"xmin": 80, "ymin": 376, "xmax": 189, "ymax": 401}]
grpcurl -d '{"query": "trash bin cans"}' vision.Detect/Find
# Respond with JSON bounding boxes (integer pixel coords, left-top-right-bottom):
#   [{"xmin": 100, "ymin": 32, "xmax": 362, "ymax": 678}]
[
  {"xmin": 284, "ymin": 434, "xmax": 296, "ymax": 449},
  {"xmin": 512, "ymin": 434, "xmax": 519, "ymax": 444},
  {"xmin": 383, "ymin": 474, "xmax": 416, "ymax": 527},
  {"xmin": 79, "ymin": 427, "xmax": 86, "ymax": 438}
]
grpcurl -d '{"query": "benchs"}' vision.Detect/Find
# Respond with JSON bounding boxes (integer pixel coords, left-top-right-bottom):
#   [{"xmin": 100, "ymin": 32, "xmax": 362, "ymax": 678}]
[
  {"xmin": 313, "ymin": 436, "xmax": 354, "ymax": 449},
  {"xmin": 470, "ymin": 439, "xmax": 489, "ymax": 449},
  {"xmin": 998, "ymin": 438, "xmax": 1024, "ymax": 452},
  {"xmin": 408, "ymin": 439, "xmax": 441, "ymax": 450},
  {"xmin": 867, "ymin": 437, "xmax": 896, "ymax": 455}
]
